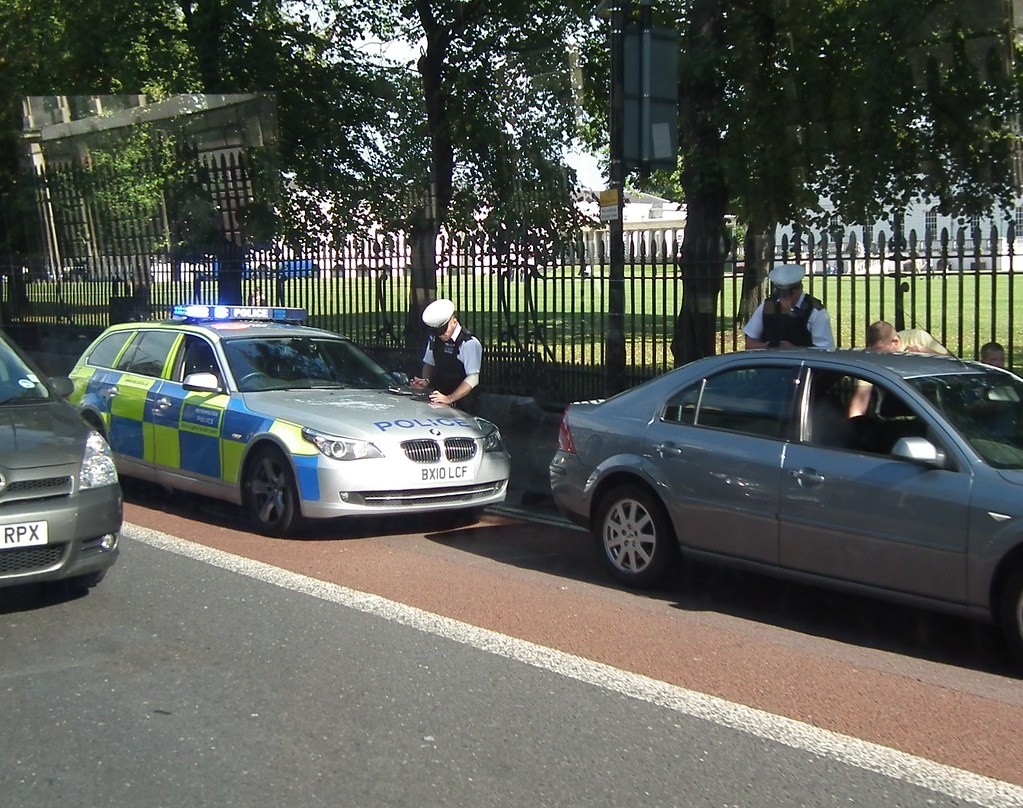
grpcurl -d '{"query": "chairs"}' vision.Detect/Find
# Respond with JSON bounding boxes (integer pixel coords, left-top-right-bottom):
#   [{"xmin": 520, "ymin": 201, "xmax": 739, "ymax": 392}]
[
  {"xmin": 807, "ymin": 391, "xmax": 854, "ymax": 455},
  {"xmin": 882, "ymin": 389, "xmax": 917, "ymax": 463}
]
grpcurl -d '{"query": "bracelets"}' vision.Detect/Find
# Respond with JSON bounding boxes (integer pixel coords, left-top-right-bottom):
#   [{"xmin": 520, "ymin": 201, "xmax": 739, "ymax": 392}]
[{"xmin": 447, "ymin": 394, "xmax": 457, "ymax": 409}]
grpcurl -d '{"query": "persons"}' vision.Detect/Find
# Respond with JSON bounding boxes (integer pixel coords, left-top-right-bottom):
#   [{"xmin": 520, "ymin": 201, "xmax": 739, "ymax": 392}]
[
  {"xmin": 825, "ymin": 262, "xmax": 951, "ymax": 281},
  {"xmin": 979, "ymin": 341, "xmax": 1006, "ymax": 370},
  {"xmin": 741, "ymin": 264, "xmax": 837, "ymax": 350},
  {"xmin": 249, "ymin": 287, "xmax": 267, "ymax": 306},
  {"xmin": 506, "ymin": 260, "xmax": 527, "ymax": 283},
  {"xmin": 845, "ymin": 320, "xmax": 952, "ymax": 420},
  {"xmin": 407, "ymin": 298, "xmax": 486, "ymax": 418}
]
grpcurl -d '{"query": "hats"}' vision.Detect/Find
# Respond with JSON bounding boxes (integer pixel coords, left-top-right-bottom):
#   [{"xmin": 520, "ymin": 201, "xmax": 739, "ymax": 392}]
[
  {"xmin": 769, "ymin": 263, "xmax": 805, "ymax": 291},
  {"xmin": 422, "ymin": 298, "xmax": 455, "ymax": 337}
]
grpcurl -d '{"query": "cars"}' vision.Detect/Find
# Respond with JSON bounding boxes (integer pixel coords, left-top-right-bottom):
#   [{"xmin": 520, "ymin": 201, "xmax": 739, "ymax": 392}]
[
  {"xmin": 1, "ymin": 330, "xmax": 121, "ymax": 590},
  {"xmin": 68, "ymin": 302, "xmax": 510, "ymax": 536},
  {"xmin": 548, "ymin": 345, "xmax": 1023, "ymax": 639}
]
